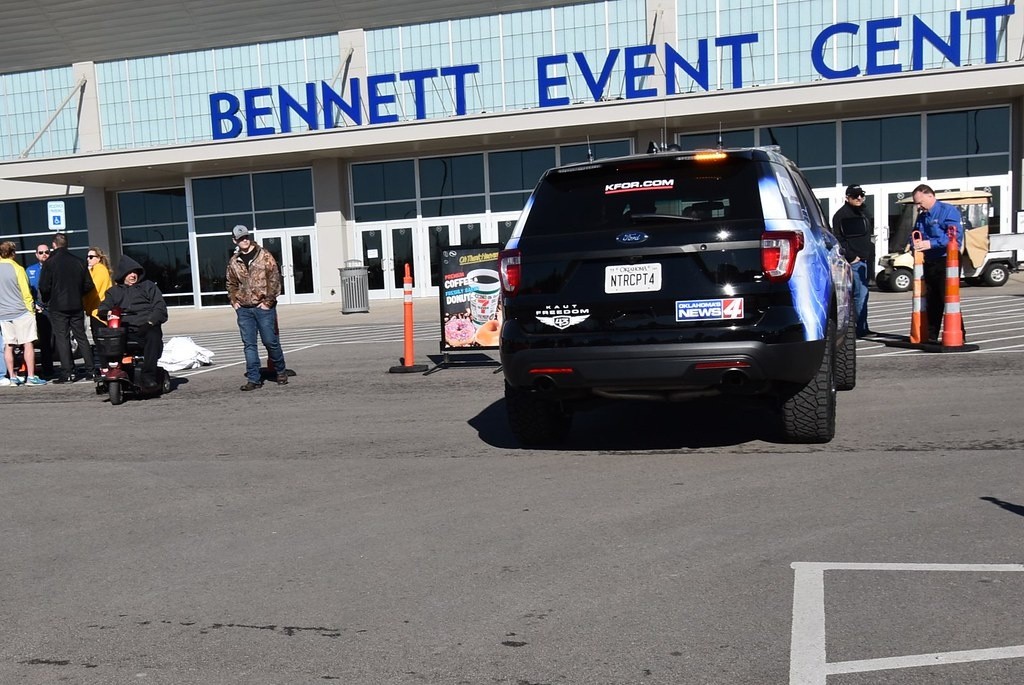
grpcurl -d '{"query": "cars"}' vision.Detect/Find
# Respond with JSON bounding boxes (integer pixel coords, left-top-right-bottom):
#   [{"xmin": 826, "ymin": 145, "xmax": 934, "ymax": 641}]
[{"xmin": 874, "ymin": 187, "xmax": 1024, "ymax": 292}]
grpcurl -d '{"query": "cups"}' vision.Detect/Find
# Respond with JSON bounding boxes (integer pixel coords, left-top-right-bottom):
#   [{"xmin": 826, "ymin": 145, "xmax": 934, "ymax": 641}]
[{"xmin": 467, "ymin": 268, "xmax": 501, "ymax": 329}]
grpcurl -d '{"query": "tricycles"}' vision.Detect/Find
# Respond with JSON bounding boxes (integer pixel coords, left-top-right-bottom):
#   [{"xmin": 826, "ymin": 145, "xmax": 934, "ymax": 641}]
[{"xmin": 92, "ymin": 309, "xmax": 171, "ymax": 405}]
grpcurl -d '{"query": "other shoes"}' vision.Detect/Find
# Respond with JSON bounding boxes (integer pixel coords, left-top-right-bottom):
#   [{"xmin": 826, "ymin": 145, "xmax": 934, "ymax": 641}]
[
  {"xmin": 52, "ymin": 376, "xmax": 73, "ymax": 384},
  {"xmin": 858, "ymin": 330, "xmax": 877, "ymax": 337},
  {"xmin": 86, "ymin": 373, "xmax": 96, "ymax": 380}
]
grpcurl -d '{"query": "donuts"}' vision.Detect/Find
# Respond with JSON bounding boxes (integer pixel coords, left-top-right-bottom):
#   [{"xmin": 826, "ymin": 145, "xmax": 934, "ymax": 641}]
[{"xmin": 446, "ymin": 319, "xmax": 502, "ymax": 347}]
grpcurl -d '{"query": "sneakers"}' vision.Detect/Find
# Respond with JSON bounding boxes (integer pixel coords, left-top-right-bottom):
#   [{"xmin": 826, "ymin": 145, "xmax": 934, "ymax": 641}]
[
  {"xmin": 10, "ymin": 377, "xmax": 24, "ymax": 387},
  {"xmin": 0, "ymin": 377, "xmax": 9, "ymax": 386},
  {"xmin": 25, "ymin": 375, "xmax": 47, "ymax": 386}
]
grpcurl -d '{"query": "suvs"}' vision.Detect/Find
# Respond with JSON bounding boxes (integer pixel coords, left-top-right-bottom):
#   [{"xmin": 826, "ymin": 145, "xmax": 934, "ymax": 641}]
[{"xmin": 497, "ymin": 144, "xmax": 858, "ymax": 443}]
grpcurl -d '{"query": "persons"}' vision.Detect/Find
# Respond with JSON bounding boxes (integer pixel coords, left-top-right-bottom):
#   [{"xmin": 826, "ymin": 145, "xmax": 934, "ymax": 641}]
[
  {"xmin": 98, "ymin": 254, "xmax": 170, "ymax": 389},
  {"xmin": 0, "ymin": 327, "xmax": 26, "ymax": 387},
  {"xmin": 833, "ymin": 185, "xmax": 880, "ymax": 339},
  {"xmin": 1, "ymin": 241, "xmax": 48, "ymax": 387},
  {"xmin": 79, "ymin": 246, "xmax": 115, "ymax": 372},
  {"xmin": 908, "ymin": 185, "xmax": 967, "ymax": 347},
  {"xmin": 38, "ymin": 234, "xmax": 103, "ymax": 385},
  {"xmin": 226, "ymin": 225, "xmax": 290, "ymax": 391},
  {"xmin": 25, "ymin": 243, "xmax": 65, "ymax": 386}
]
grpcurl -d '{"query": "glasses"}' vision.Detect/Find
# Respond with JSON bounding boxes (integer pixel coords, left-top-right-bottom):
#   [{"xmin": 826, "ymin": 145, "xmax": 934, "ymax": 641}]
[
  {"xmin": 86, "ymin": 255, "xmax": 98, "ymax": 259},
  {"xmin": 37, "ymin": 250, "xmax": 49, "ymax": 254}
]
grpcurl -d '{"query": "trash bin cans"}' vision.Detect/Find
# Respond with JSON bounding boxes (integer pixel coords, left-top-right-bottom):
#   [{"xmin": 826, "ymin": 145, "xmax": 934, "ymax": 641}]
[{"xmin": 337, "ymin": 259, "xmax": 369, "ymax": 314}]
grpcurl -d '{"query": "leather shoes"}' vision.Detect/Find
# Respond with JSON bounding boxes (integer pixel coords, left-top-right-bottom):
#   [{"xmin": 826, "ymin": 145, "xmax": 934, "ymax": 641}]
[
  {"xmin": 240, "ymin": 382, "xmax": 263, "ymax": 390},
  {"xmin": 141, "ymin": 378, "xmax": 157, "ymax": 388},
  {"xmin": 278, "ymin": 372, "xmax": 288, "ymax": 385}
]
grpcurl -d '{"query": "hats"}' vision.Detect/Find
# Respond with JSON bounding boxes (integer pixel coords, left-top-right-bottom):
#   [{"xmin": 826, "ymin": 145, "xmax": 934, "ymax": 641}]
[
  {"xmin": 232, "ymin": 224, "xmax": 250, "ymax": 240},
  {"xmin": 846, "ymin": 184, "xmax": 865, "ymax": 196}
]
